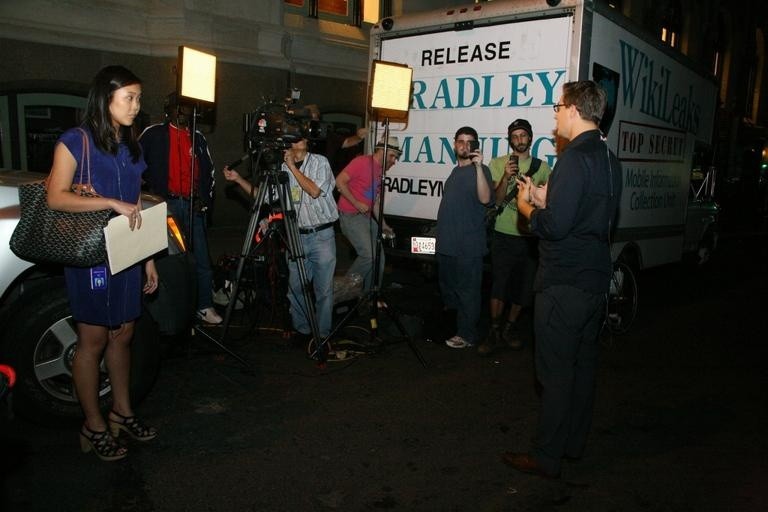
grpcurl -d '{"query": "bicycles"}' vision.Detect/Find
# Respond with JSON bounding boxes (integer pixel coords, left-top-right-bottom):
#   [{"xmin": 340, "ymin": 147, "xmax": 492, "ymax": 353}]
[{"xmin": 605, "ymin": 257, "xmax": 643, "ymax": 339}]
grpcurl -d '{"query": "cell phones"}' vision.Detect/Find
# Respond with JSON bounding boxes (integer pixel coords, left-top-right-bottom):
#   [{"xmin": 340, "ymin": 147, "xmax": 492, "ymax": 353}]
[
  {"xmin": 510, "ymin": 155, "xmax": 518, "ymax": 172},
  {"xmin": 470, "ymin": 141, "xmax": 479, "ymax": 159}
]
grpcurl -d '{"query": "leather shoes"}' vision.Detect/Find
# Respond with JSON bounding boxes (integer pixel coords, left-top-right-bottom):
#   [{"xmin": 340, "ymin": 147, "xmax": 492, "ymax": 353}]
[{"xmin": 510, "ymin": 450, "xmax": 568, "ymax": 482}]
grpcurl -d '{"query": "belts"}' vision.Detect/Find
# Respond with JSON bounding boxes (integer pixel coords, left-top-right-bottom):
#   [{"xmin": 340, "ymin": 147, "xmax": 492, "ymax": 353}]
[{"xmin": 297, "ymin": 222, "xmax": 335, "ymax": 233}]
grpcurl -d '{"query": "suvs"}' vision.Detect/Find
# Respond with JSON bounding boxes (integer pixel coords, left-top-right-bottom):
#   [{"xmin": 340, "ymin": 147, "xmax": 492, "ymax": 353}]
[{"xmin": 0, "ymin": 167, "xmax": 198, "ymax": 425}]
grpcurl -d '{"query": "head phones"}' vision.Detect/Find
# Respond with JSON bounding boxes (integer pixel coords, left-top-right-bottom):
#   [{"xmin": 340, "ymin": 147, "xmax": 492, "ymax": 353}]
[{"xmin": 165, "ymin": 92, "xmax": 202, "ymax": 123}]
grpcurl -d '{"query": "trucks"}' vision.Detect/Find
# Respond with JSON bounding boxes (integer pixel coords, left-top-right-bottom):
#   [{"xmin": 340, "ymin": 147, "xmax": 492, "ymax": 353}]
[{"xmin": 361, "ymin": 2, "xmax": 731, "ymax": 322}]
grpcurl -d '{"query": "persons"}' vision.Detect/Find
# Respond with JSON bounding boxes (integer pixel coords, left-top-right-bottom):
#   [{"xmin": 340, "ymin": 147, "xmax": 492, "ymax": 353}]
[
  {"xmin": 223, "ymin": 134, "xmax": 338, "ymax": 361},
  {"xmin": 435, "ymin": 125, "xmax": 495, "ymax": 350},
  {"xmin": 516, "ymin": 81, "xmax": 621, "ymax": 486},
  {"xmin": 135, "ymin": 89, "xmax": 224, "ymax": 326},
  {"xmin": 336, "ymin": 137, "xmax": 401, "ymax": 308},
  {"xmin": 489, "ymin": 118, "xmax": 550, "ymax": 349},
  {"xmin": 46, "ymin": 66, "xmax": 159, "ymax": 462}
]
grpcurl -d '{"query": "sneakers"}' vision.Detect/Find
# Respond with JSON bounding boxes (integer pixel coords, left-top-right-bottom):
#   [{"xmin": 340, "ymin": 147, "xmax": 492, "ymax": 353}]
[
  {"xmin": 445, "ymin": 335, "xmax": 475, "ymax": 349},
  {"xmin": 193, "ymin": 307, "xmax": 224, "ymax": 325}
]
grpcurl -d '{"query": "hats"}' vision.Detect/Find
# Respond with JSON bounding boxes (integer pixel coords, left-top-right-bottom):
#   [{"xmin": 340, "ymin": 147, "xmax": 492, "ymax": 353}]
[
  {"xmin": 507, "ymin": 119, "xmax": 534, "ymax": 137},
  {"xmin": 281, "ymin": 130, "xmax": 304, "ymax": 142},
  {"xmin": 375, "ymin": 137, "xmax": 404, "ymax": 155}
]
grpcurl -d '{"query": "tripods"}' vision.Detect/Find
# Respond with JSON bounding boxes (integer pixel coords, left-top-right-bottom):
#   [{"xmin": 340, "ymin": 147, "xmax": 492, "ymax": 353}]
[
  {"xmin": 219, "ymin": 169, "xmax": 327, "ymax": 370},
  {"xmin": 311, "ymin": 116, "xmax": 429, "ymax": 369},
  {"xmin": 157, "ymin": 102, "xmax": 255, "ymax": 372}
]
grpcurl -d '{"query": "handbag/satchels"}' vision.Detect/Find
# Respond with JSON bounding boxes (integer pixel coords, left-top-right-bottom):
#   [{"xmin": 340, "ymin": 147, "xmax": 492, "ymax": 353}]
[{"xmin": 9, "ymin": 179, "xmax": 113, "ymax": 268}]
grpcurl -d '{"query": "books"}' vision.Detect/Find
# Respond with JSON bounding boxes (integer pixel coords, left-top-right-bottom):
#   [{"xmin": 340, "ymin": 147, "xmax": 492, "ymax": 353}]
[{"xmin": 101, "ymin": 200, "xmax": 168, "ymax": 276}]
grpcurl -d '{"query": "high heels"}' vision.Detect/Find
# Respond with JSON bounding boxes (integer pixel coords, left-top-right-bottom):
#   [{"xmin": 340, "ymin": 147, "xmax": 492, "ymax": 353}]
[
  {"xmin": 78, "ymin": 421, "xmax": 128, "ymax": 461},
  {"xmin": 108, "ymin": 408, "xmax": 158, "ymax": 442}
]
